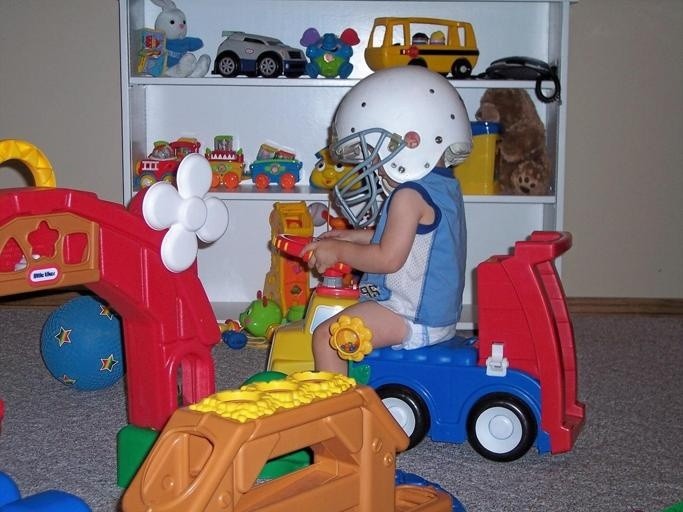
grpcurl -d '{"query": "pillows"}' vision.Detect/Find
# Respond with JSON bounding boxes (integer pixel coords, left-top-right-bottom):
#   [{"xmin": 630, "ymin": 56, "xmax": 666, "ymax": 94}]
[{"xmin": 329, "ymin": 65, "xmax": 473, "ymax": 229}]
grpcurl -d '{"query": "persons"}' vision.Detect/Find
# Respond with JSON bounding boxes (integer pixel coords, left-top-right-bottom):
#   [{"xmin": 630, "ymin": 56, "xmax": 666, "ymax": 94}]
[{"xmin": 297, "ymin": 63, "xmax": 473, "ymax": 382}]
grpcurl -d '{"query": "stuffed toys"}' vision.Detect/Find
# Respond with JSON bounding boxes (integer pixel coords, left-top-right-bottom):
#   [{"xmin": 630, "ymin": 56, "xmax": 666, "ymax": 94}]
[
  {"xmin": 475, "ymin": 88, "xmax": 555, "ymax": 197},
  {"xmin": 150, "ymin": 0, "xmax": 211, "ymax": 78}
]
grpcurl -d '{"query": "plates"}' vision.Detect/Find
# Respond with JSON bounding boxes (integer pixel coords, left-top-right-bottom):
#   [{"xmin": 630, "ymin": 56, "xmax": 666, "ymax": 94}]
[{"xmin": 118, "ymin": 0, "xmax": 571, "ymax": 331}]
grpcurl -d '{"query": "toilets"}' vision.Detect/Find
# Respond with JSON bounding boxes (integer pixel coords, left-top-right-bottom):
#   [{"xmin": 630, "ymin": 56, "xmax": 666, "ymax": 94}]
[{"xmin": 486, "ymin": 56, "xmax": 552, "ymax": 80}]
